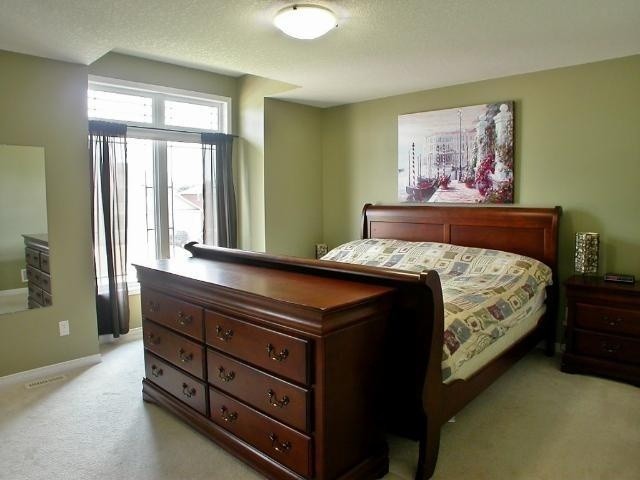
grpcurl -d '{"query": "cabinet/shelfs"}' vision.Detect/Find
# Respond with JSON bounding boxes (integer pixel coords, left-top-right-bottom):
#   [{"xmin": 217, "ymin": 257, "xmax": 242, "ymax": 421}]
[
  {"xmin": 130, "ymin": 252, "xmax": 394, "ymax": 480},
  {"xmin": 18, "ymin": 230, "xmax": 54, "ymax": 311}
]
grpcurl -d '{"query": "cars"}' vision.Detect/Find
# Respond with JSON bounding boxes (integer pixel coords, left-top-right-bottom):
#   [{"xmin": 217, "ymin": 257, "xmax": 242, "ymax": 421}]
[{"xmin": 140, "ymin": 183, "xmax": 204, "ymax": 247}]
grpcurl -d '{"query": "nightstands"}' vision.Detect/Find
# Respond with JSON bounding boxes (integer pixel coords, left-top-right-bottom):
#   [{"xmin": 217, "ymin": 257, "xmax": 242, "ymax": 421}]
[{"xmin": 559, "ymin": 270, "xmax": 639, "ymax": 390}]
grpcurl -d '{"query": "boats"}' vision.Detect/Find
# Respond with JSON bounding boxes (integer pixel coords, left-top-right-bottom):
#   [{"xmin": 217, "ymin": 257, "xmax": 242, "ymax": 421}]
[{"xmin": 398, "ymin": 141, "xmax": 468, "ymax": 197}]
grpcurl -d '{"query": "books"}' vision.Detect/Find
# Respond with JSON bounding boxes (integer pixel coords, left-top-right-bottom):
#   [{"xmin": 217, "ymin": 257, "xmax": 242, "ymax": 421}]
[{"xmin": 604, "ymin": 272, "xmax": 634, "ymax": 283}]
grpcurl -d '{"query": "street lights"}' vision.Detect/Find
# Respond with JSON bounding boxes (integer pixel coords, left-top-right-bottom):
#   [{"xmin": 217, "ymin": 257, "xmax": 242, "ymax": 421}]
[{"xmin": 456, "ymin": 109, "xmax": 463, "ymax": 180}]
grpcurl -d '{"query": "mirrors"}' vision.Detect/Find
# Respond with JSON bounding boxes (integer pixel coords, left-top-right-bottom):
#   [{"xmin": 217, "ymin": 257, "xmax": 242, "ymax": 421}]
[{"xmin": 1, "ymin": 144, "xmax": 54, "ymax": 318}]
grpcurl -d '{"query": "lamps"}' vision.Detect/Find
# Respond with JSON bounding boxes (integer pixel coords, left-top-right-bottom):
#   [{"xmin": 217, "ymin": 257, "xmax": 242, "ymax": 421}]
[
  {"xmin": 572, "ymin": 229, "xmax": 601, "ymax": 287},
  {"xmin": 269, "ymin": 1, "xmax": 339, "ymax": 42}
]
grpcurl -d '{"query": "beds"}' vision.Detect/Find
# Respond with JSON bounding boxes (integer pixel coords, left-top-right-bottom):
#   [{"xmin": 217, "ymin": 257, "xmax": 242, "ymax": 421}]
[{"xmin": 184, "ymin": 198, "xmax": 568, "ymax": 479}]
